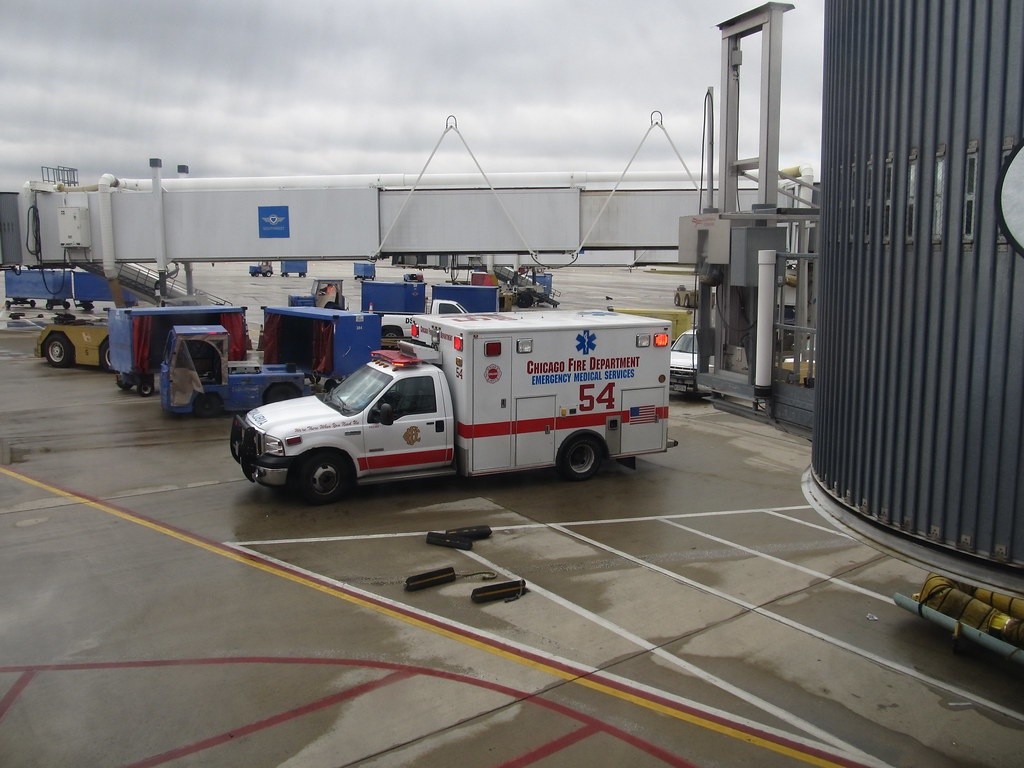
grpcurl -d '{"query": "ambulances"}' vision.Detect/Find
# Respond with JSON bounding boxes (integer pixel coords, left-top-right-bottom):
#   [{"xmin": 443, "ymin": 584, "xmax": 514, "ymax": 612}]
[{"xmin": 229, "ymin": 308, "xmax": 679, "ymax": 507}]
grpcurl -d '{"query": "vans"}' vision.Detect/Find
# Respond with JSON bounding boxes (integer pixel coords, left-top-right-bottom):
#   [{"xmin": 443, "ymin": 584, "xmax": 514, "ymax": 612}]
[{"xmin": 668, "ymin": 330, "xmax": 716, "ymax": 407}]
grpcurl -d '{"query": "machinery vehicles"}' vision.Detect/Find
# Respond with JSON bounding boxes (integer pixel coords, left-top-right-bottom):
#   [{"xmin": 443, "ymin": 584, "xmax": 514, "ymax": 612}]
[{"xmin": 249, "ymin": 259, "xmax": 273, "ymax": 277}]
